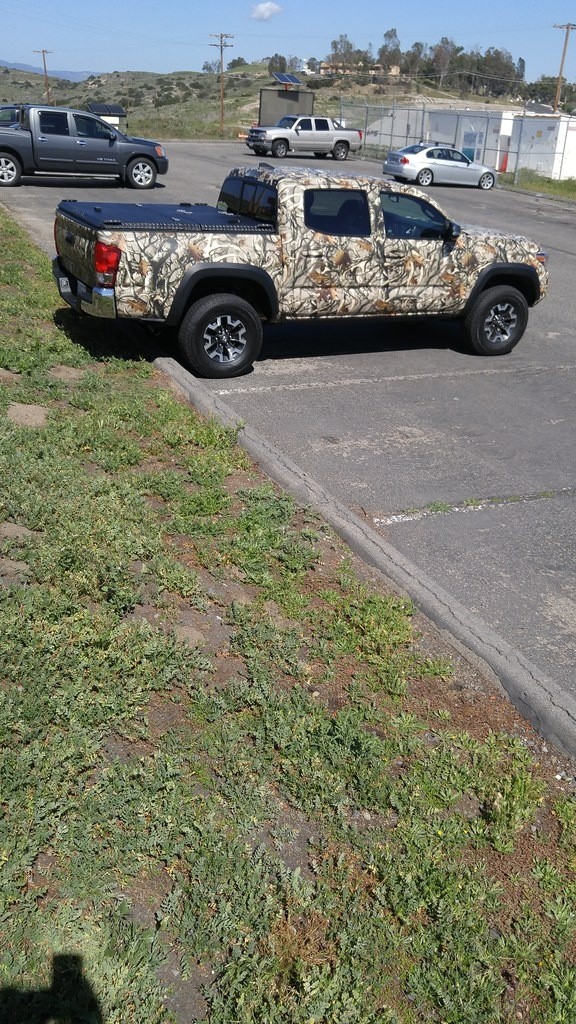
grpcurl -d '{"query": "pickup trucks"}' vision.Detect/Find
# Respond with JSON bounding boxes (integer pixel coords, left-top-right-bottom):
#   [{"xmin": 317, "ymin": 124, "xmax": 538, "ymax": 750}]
[
  {"xmin": 0, "ymin": 106, "xmax": 169, "ymax": 189},
  {"xmin": 52, "ymin": 161, "xmax": 550, "ymax": 380},
  {"xmin": 245, "ymin": 114, "xmax": 364, "ymax": 161}
]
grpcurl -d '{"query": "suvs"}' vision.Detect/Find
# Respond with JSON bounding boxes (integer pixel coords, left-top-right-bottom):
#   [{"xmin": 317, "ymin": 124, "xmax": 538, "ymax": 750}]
[{"xmin": 0, "ymin": 105, "xmax": 95, "ymax": 135}]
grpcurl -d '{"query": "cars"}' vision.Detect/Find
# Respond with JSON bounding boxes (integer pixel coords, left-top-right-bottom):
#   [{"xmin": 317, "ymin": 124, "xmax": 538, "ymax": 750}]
[{"xmin": 382, "ymin": 146, "xmax": 498, "ymax": 190}]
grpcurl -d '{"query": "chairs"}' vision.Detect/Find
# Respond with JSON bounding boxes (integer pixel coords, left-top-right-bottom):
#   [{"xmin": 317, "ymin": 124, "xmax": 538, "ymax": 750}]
[
  {"xmin": 332, "ymin": 198, "xmax": 367, "ymax": 237},
  {"xmin": 437, "ymin": 153, "xmax": 445, "ymax": 160}
]
